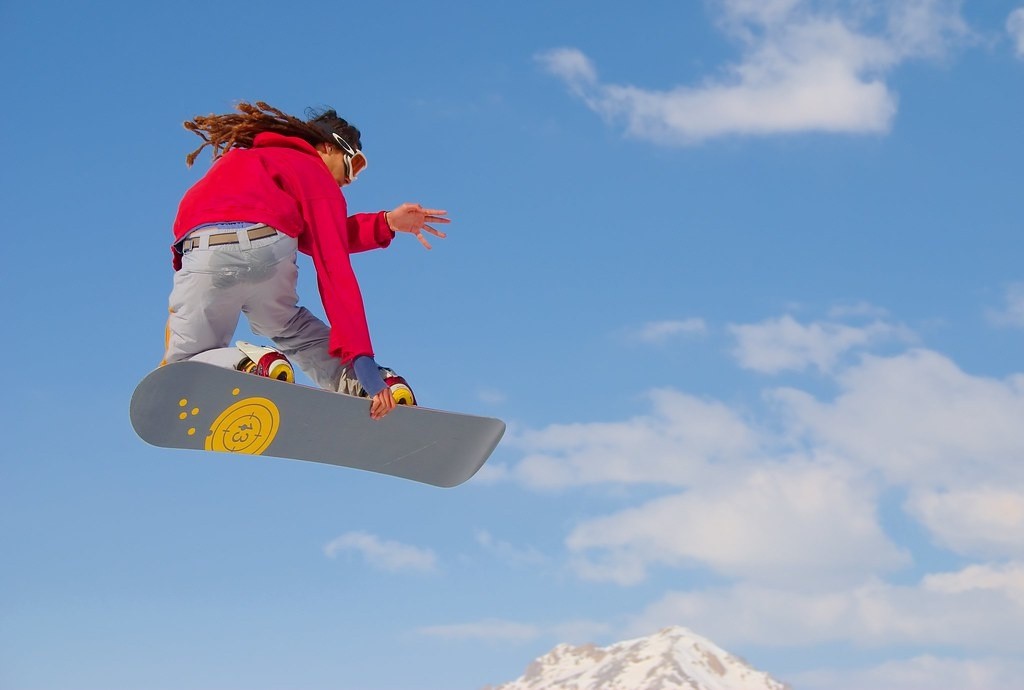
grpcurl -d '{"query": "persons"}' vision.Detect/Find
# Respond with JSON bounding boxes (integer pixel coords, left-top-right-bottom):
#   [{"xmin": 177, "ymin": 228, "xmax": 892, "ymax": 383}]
[{"xmin": 158, "ymin": 96, "xmax": 451, "ymax": 422}]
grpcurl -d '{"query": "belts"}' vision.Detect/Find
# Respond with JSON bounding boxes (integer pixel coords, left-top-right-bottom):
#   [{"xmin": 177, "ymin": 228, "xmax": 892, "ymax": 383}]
[{"xmin": 182, "ymin": 225, "xmax": 277, "ymax": 255}]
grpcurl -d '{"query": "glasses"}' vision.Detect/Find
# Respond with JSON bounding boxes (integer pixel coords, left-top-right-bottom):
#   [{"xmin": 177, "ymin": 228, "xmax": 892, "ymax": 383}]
[{"xmin": 345, "ymin": 150, "xmax": 368, "ymax": 179}]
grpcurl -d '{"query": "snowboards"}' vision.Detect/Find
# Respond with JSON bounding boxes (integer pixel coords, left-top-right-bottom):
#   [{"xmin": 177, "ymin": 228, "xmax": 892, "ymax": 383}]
[{"xmin": 130, "ymin": 360, "xmax": 506, "ymax": 489}]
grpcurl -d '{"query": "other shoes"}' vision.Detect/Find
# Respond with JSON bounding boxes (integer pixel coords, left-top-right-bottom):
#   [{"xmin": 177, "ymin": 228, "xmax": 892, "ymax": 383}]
[
  {"xmin": 364, "ymin": 367, "xmax": 416, "ymax": 406},
  {"xmin": 236, "ymin": 340, "xmax": 294, "ymax": 382}
]
